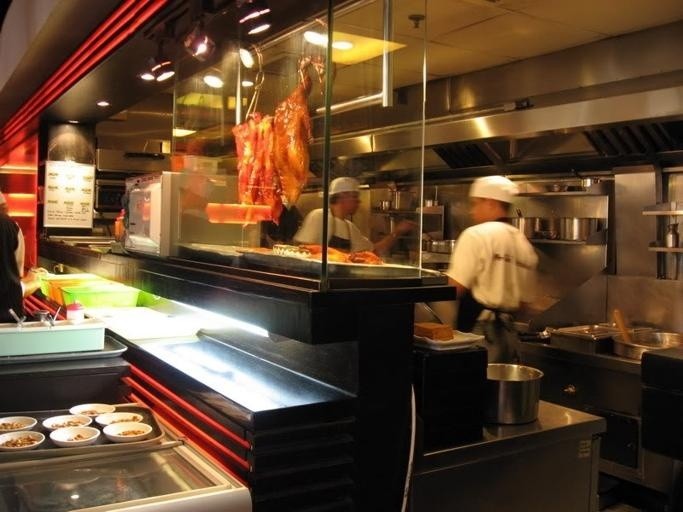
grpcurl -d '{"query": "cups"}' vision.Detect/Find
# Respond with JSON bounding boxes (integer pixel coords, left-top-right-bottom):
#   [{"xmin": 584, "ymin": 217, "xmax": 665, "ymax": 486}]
[{"xmin": 378, "ymin": 199, "xmax": 390, "ymax": 211}]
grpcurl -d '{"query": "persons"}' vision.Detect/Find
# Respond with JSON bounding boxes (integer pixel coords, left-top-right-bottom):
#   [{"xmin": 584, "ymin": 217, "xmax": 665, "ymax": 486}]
[
  {"xmin": 440, "ymin": 175, "xmax": 540, "ymax": 366},
  {"xmin": 0, "ymin": 190, "xmax": 27, "ymax": 325},
  {"xmin": 289, "ymin": 177, "xmax": 419, "ymax": 255}
]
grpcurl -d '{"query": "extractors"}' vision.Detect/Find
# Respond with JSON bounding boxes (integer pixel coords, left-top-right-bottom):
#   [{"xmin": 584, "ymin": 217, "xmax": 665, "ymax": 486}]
[{"xmin": 579, "ymin": 120, "xmax": 682, "ymax": 162}]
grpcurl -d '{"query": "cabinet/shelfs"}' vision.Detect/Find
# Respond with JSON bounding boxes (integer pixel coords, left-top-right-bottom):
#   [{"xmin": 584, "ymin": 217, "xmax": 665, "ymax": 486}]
[
  {"xmin": 373, "ymin": 200, "xmax": 444, "ymax": 246},
  {"xmin": 514, "ymin": 175, "xmax": 610, "ymax": 254},
  {"xmin": 638, "ymin": 198, "xmax": 681, "ymax": 256}
]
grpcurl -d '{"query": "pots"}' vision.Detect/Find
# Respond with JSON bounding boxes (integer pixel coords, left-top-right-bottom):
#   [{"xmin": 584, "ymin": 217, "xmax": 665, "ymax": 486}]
[
  {"xmin": 485, "ymin": 360, "xmax": 543, "ymax": 426},
  {"xmin": 102, "ymin": 421, "xmax": 152, "ymax": 442},
  {"xmin": 558, "ymin": 215, "xmax": 607, "ymax": 241},
  {"xmin": 506, "ymin": 215, "xmax": 541, "ymax": 239}
]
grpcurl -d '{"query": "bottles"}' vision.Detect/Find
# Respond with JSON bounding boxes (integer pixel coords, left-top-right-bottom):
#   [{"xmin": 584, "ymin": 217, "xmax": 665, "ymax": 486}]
[
  {"xmin": 113, "ymin": 215, "xmax": 125, "ymax": 242},
  {"xmin": 663, "ymin": 224, "xmax": 679, "ymax": 247},
  {"xmin": 65, "ymin": 299, "xmax": 85, "ymax": 320}
]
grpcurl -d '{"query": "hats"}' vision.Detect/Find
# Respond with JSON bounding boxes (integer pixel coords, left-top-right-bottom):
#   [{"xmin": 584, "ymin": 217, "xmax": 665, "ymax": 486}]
[
  {"xmin": 328, "ymin": 176, "xmax": 361, "ymax": 194},
  {"xmin": 468, "ymin": 175, "xmax": 517, "ymax": 203}
]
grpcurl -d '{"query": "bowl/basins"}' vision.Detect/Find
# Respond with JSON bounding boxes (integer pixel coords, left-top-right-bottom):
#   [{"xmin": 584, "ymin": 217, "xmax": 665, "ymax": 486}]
[
  {"xmin": 417, "ymin": 199, "xmax": 438, "ymax": 209},
  {"xmin": 47, "ymin": 426, "xmax": 100, "ymax": 447},
  {"xmin": 425, "ymin": 240, "xmax": 455, "ymax": 254},
  {"xmin": 579, "ymin": 176, "xmax": 599, "ymax": 191},
  {"xmin": 0, "ymin": 415, "xmax": 37, "ymax": 433},
  {"xmin": 68, "ymin": 403, "xmax": 116, "ymax": 422},
  {"xmin": 542, "ymin": 183, "xmax": 567, "ymax": 192},
  {"xmin": 0, "ymin": 430, "xmax": 45, "ymax": 451},
  {"xmin": 40, "ymin": 415, "xmax": 92, "ymax": 433},
  {"xmin": 94, "ymin": 412, "xmax": 144, "ymax": 428}
]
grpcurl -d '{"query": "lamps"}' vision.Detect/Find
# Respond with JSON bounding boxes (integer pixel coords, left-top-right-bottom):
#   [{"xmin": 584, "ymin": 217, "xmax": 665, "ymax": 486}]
[{"xmin": 135, "ymin": 0, "xmax": 270, "ymax": 89}]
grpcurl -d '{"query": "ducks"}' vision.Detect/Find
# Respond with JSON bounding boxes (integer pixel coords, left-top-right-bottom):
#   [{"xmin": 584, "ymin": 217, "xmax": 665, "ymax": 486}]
[{"xmin": 271, "ymin": 51, "xmax": 336, "ymax": 211}]
